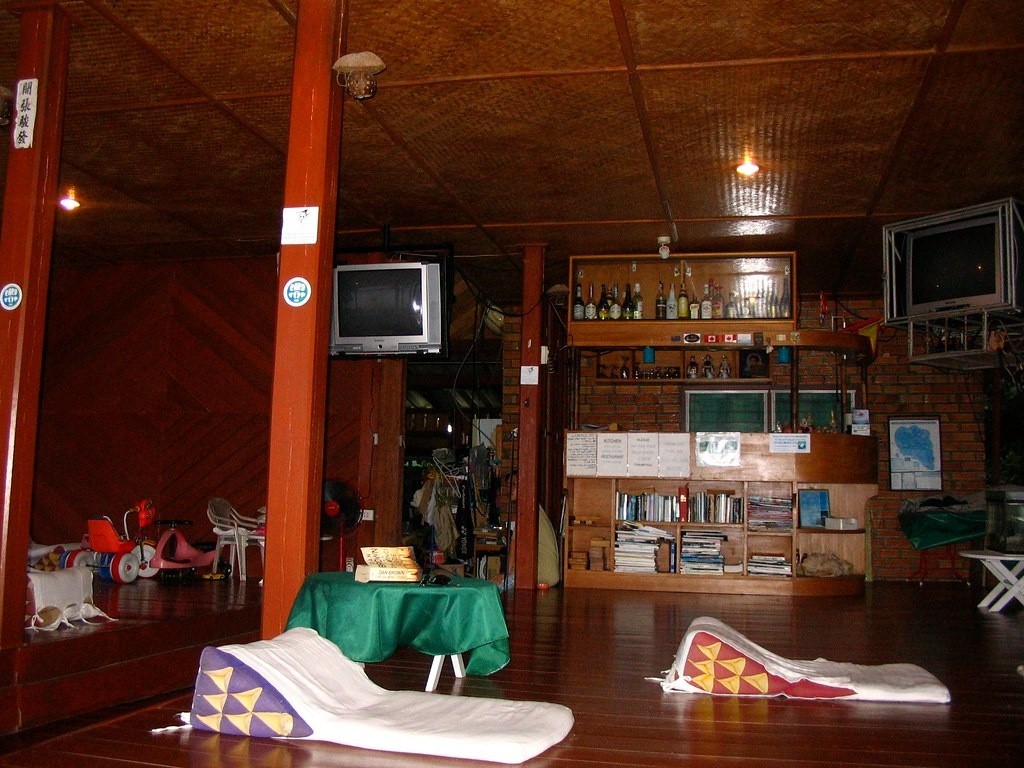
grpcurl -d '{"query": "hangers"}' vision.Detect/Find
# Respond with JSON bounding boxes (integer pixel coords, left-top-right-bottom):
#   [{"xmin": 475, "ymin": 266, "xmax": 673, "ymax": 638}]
[{"xmin": 432, "ymin": 456, "xmax": 462, "ymax": 498}]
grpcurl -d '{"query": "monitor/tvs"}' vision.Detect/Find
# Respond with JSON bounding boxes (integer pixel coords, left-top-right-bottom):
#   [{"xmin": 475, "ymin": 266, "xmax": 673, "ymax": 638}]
[
  {"xmin": 328, "ymin": 262, "xmax": 441, "ymax": 354},
  {"xmin": 905, "ymin": 216, "xmax": 1003, "ymax": 317}
]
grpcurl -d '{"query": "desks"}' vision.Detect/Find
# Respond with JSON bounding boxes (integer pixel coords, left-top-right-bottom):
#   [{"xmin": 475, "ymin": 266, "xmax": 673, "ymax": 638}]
[
  {"xmin": 286, "ymin": 571, "xmax": 510, "ymax": 693},
  {"xmin": 958, "ymin": 550, "xmax": 1024, "ymax": 612}
]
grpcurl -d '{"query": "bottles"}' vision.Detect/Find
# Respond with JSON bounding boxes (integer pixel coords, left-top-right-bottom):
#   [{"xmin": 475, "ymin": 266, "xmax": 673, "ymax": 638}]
[
  {"xmin": 598, "ymin": 285, "xmax": 611, "ymax": 320},
  {"xmin": 656, "ymin": 280, "xmax": 666, "ymax": 320},
  {"xmin": 677, "ymin": 282, "xmax": 690, "ymax": 320},
  {"xmin": 609, "ymin": 283, "xmax": 623, "ymax": 320},
  {"xmin": 573, "ymin": 285, "xmax": 585, "ymax": 321},
  {"xmin": 585, "ymin": 284, "xmax": 598, "ymax": 320},
  {"xmin": 632, "ymin": 282, "xmax": 644, "ymax": 320},
  {"xmin": 621, "ymin": 283, "xmax": 635, "ymax": 320},
  {"xmin": 666, "ymin": 283, "xmax": 678, "ymax": 320},
  {"xmin": 606, "ymin": 286, "xmax": 613, "ymax": 306},
  {"xmin": 619, "ymin": 356, "xmax": 680, "ymax": 379},
  {"xmin": 689, "ymin": 277, "xmax": 791, "ymax": 319}
]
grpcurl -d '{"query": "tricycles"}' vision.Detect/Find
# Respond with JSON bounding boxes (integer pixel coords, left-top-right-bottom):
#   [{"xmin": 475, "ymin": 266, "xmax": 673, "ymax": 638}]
[{"xmin": 60, "ymin": 498, "xmax": 163, "ymax": 584}]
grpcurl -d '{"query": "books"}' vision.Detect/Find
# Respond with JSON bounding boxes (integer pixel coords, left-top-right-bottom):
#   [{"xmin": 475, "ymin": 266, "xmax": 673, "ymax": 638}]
[
  {"xmin": 747, "ymin": 553, "xmax": 792, "ymax": 578},
  {"xmin": 615, "ymin": 486, "xmax": 689, "ymax": 523},
  {"xmin": 614, "ymin": 520, "xmax": 676, "ymax": 573},
  {"xmin": 680, "ymin": 529, "xmax": 743, "ymax": 575},
  {"xmin": 747, "ymin": 495, "xmax": 792, "ymax": 528},
  {"xmin": 689, "ymin": 489, "xmax": 744, "ymax": 524}
]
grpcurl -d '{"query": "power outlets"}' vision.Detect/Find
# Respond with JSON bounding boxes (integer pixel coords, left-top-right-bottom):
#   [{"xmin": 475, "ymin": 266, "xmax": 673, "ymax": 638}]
[{"xmin": 362, "ymin": 510, "xmax": 374, "ymax": 522}]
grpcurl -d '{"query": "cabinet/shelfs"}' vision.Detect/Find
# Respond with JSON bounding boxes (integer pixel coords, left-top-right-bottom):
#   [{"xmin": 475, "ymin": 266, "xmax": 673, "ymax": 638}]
[
  {"xmin": 569, "ymin": 251, "xmax": 796, "ymax": 382},
  {"xmin": 564, "ymin": 475, "xmax": 877, "ymax": 596}
]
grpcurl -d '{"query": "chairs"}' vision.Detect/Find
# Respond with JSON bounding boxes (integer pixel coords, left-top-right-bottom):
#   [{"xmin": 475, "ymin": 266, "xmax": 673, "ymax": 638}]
[{"xmin": 207, "ymin": 497, "xmax": 266, "ymax": 581}]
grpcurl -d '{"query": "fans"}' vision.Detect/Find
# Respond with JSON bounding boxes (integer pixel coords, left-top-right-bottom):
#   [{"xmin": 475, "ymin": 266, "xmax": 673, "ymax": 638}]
[{"xmin": 321, "ymin": 478, "xmax": 365, "ymax": 573}]
